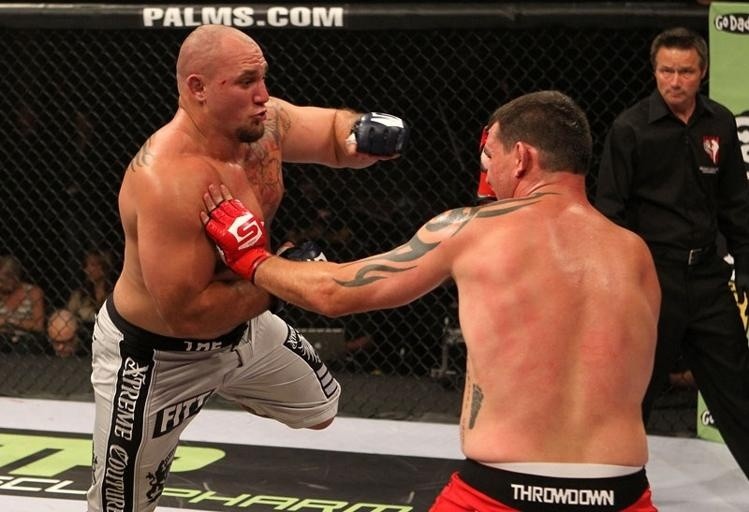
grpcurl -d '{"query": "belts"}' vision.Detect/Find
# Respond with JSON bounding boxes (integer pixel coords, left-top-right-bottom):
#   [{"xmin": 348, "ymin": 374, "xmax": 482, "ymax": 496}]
[{"xmin": 660, "ymin": 247, "xmax": 717, "ymax": 266}]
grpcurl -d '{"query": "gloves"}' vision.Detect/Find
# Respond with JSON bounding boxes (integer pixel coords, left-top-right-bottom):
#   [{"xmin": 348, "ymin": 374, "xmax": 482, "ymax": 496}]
[
  {"xmin": 346, "ymin": 112, "xmax": 408, "ymax": 157},
  {"xmin": 203, "ymin": 198, "xmax": 271, "ymax": 283},
  {"xmin": 277, "ymin": 241, "xmax": 327, "ymax": 262},
  {"xmin": 478, "ymin": 127, "xmax": 499, "ymax": 199}
]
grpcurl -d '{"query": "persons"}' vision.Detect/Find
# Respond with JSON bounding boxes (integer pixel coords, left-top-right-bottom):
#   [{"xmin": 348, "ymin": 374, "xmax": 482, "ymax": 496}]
[
  {"xmin": 85, "ymin": 23, "xmax": 406, "ymax": 512},
  {"xmin": 595, "ymin": 27, "xmax": 749, "ymax": 481},
  {"xmin": 1, "ymin": 105, "xmax": 371, "ymax": 380},
  {"xmin": 199, "ymin": 88, "xmax": 662, "ymax": 511}
]
